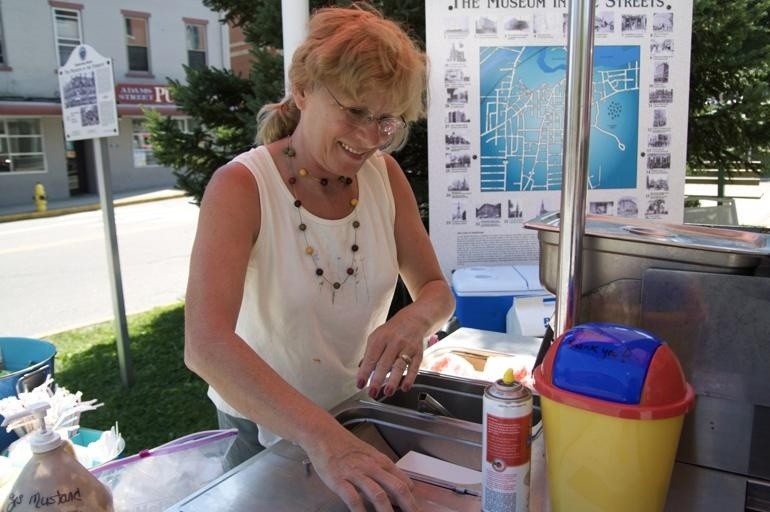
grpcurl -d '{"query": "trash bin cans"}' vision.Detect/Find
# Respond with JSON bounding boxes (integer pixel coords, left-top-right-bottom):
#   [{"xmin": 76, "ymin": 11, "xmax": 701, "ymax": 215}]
[{"xmin": 530, "ymin": 321, "xmax": 695, "ymax": 510}]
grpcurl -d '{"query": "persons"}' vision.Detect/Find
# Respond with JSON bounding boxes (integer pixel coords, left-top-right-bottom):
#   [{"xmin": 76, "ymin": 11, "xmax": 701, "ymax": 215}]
[{"xmin": 180, "ymin": 4, "xmax": 459, "ymax": 510}]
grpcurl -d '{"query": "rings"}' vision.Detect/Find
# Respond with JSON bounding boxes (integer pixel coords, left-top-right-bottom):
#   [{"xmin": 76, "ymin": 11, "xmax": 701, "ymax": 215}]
[{"xmin": 397, "ymin": 355, "xmax": 415, "ymax": 365}]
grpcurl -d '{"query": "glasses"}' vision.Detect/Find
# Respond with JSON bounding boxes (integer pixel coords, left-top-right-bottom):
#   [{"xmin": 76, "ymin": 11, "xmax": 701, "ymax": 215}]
[{"xmin": 322, "ymin": 80, "xmax": 409, "ymax": 136}]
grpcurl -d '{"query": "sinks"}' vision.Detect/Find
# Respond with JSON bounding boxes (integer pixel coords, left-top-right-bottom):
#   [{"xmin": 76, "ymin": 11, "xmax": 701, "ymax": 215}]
[
  {"xmin": 362, "ymin": 371, "xmax": 540, "ymax": 440},
  {"xmin": 314, "ymin": 406, "xmax": 505, "ymax": 498}
]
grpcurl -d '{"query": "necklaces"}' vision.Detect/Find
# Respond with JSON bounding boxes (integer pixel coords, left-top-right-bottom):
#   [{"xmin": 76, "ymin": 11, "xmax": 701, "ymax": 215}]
[{"xmin": 281, "ymin": 134, "xmax": 364, "ymax": 302}]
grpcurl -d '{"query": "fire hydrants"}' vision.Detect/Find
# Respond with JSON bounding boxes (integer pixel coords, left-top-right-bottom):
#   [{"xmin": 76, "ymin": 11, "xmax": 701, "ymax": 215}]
[{"xmin": 33, "ymin": 181, "xmax": 47, "ymax": 212}]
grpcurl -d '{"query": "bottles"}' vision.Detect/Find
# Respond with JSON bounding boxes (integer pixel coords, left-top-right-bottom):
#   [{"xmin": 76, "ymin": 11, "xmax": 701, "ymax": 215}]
[{"xmin": 482, "ymin": 369, "xmax": 533, "ymax": 512}]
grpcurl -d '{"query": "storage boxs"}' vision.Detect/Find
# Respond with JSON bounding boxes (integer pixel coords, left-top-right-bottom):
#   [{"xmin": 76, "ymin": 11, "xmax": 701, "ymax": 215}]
[{"xmin": 452, "ymin": 264, "xmax": 556, "ymax": 332}]
